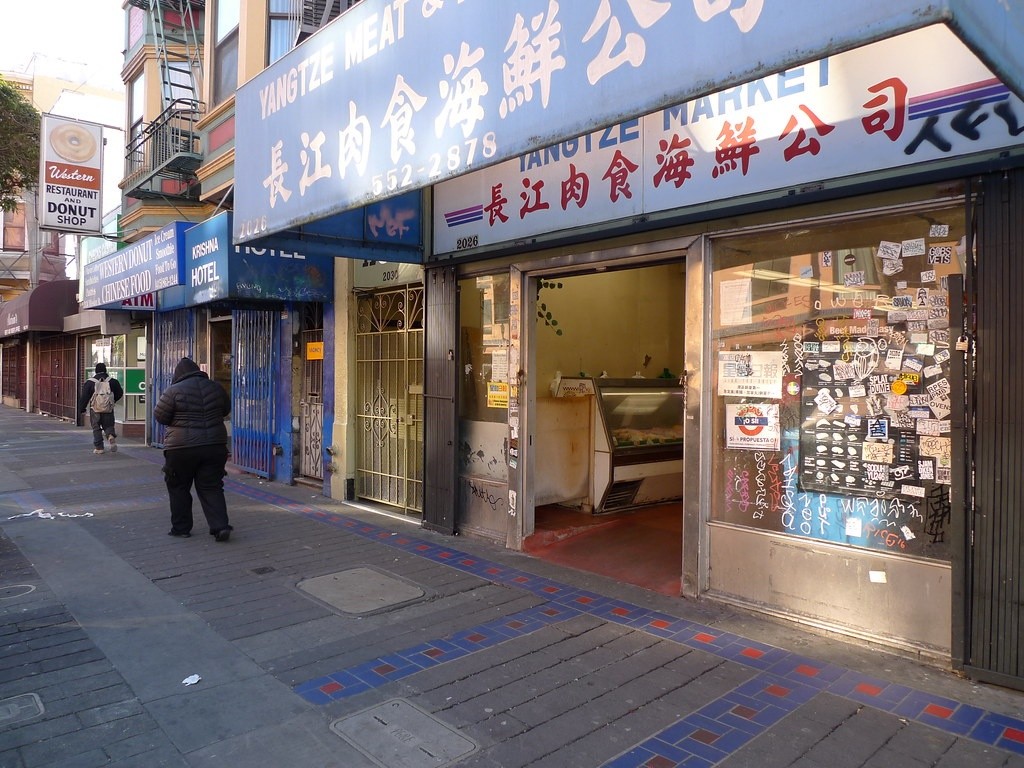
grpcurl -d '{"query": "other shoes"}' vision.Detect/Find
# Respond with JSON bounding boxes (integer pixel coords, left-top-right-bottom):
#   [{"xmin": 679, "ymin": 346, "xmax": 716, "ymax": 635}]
[
  {"xmin": 214, "ymin": 528, "xmax": 231, "ymax": 542},
  {"xmin": 169, "ymin": 528, "xmax": 191, "ymax": 538}
]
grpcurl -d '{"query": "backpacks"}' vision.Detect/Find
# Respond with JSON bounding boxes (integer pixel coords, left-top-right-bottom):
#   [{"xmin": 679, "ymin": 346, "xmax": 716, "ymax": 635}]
[{"xmin": 87, "ymin": 376, "xmax": 117, "ymax": 414}]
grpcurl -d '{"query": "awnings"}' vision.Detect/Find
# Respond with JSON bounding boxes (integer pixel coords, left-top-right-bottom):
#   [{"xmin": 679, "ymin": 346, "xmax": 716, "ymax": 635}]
[{"xmin": 0, "ymin": 280, "xmax": 77, "ymax": 337}]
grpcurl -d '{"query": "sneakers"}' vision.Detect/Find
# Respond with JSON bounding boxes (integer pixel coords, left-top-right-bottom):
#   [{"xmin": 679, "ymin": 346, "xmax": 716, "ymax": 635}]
[
  {"xmin": 93, "ymin": 449, "xmax": 104, "ymax": 453},
  {"xmin": 109, "ymin": 436, "xmax": 118, "ymax": 452}
]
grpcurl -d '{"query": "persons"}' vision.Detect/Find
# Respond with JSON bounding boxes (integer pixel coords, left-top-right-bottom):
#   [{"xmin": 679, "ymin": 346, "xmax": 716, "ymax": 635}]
[
  {"xmin": 154, "ymin": 356, "xmax": 234, "ymax": 541},
  {"xmin": 77, "ymin": 363, "xmax": 122, "ymax": 453}
]
